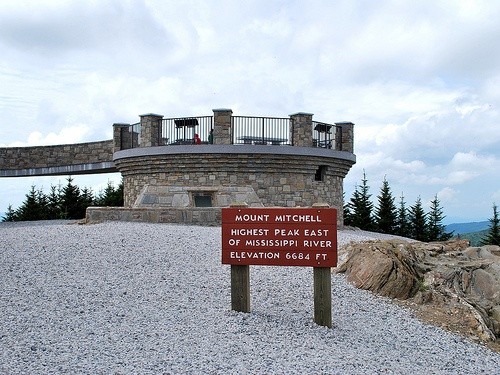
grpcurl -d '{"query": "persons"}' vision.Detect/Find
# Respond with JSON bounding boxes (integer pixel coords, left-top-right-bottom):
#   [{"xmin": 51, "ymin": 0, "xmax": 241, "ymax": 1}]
[{"xmin": 208, "ymin": 129, "xmax": 213, "ymax": 144}]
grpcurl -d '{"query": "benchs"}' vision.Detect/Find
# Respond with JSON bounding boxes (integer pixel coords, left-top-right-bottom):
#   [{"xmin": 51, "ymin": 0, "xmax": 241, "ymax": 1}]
[{"xmin": 236, "ymin": 136, "xmax": 289, "ymax": 145}]
